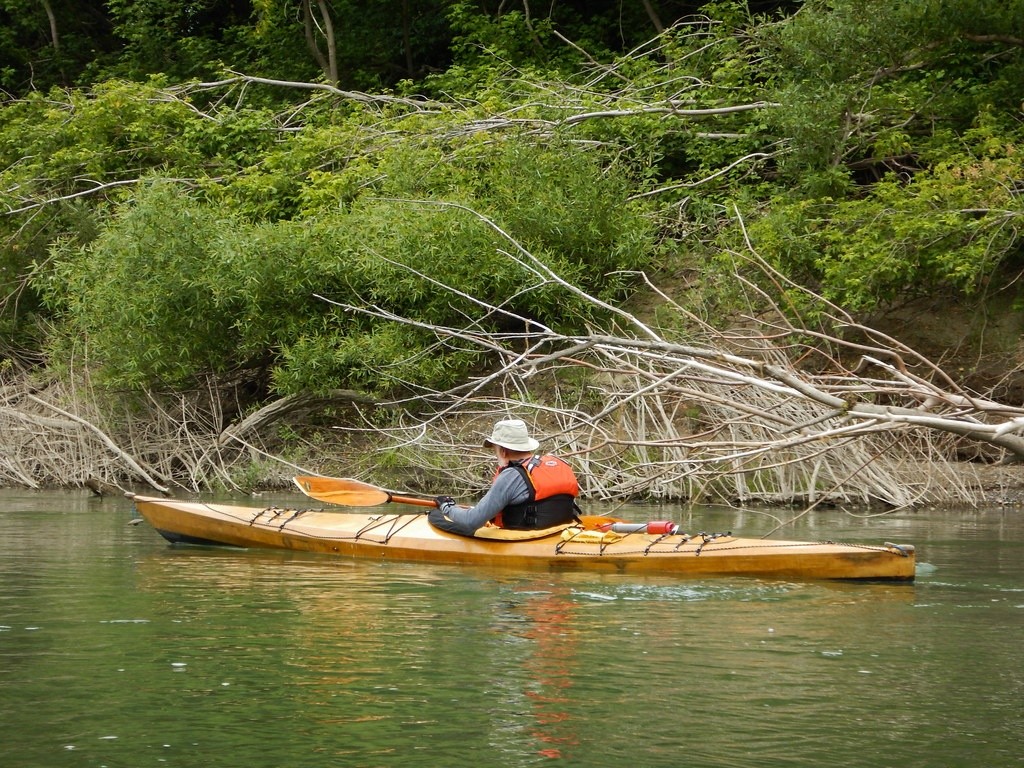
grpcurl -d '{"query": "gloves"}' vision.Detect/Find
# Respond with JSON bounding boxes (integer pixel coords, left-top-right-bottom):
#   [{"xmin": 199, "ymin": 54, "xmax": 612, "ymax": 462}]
[{"xmin": 433, "ymin": 496, "xmax": 456, "ymax": 514}]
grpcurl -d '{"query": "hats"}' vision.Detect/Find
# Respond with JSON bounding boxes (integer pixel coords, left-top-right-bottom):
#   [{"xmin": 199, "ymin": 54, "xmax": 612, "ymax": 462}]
[{"xmin": 483, "ymin": 420, "xmax": 539, "ymax": 451}]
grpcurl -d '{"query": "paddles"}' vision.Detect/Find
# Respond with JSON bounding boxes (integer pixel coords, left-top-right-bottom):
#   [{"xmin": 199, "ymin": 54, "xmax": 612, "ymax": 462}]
[{"xmin": 292, "ymin": 475, "xmax": 675, "ymax": 534}]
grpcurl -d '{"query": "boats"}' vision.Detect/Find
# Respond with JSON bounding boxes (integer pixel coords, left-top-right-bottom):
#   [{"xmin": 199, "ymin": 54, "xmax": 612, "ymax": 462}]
[{"xmin": 124, "ymin": 489, "xmax": 916, "ymax": 582}]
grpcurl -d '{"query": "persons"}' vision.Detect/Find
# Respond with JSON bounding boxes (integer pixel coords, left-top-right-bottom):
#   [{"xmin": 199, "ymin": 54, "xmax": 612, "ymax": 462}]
[{"xmin": 433, "ymin": 420, "xmax": 579, "ymax": 530}]
